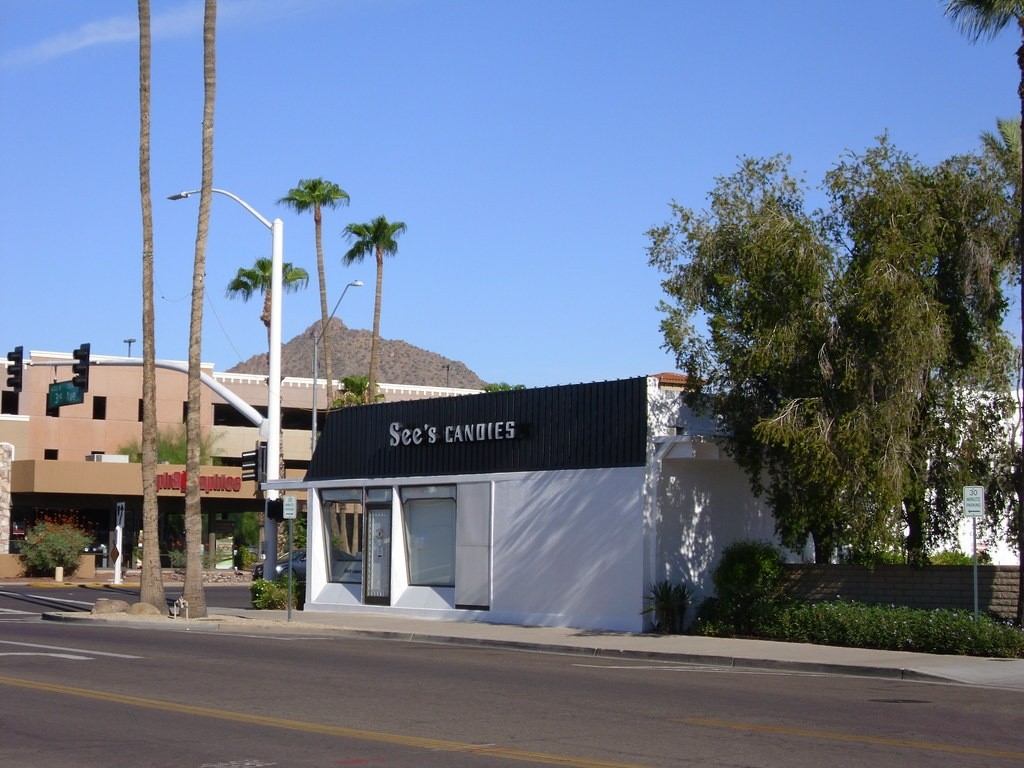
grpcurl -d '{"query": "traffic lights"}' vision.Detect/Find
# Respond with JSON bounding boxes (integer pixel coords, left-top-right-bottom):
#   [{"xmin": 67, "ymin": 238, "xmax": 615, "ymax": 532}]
[
  {"xmin": 240, "ymin": 438, "xmax": 267, "ymax": 486},
  {"xmin": 71, "ymin": 343, "xmax": 91, "ymax": 394},
  {"xmin": 6, "ymin": 343, "xmax": 25, "ymax": 394}
]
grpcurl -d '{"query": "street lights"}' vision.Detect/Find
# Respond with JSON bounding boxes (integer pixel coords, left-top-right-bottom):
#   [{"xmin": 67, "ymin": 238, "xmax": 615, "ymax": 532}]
[
  {"xmin": 165, "ymin": 189, "xmax": 283, "ymax": 582},
  {"xmin": 124, "ymin": 338, "xmax": 137, "ymax": 358},
  {"xmin": 310, "ymin": 280, "xmax": 364, "ymax": 454}
]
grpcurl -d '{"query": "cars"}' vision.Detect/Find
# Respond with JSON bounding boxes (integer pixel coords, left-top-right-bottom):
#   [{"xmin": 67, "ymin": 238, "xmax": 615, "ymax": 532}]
[{"xmin": 252, "ymin": 549, "xmax": 307, "ymax": 582}]
